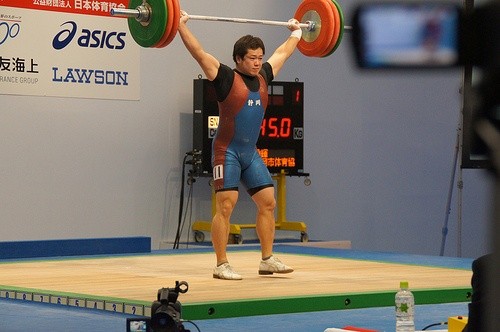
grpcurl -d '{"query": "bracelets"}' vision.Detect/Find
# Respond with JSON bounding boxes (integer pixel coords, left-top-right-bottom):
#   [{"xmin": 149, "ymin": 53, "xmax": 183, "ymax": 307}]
[{"xmin": 290, "ymin": 29, "xmax": 302, "ymax": 40}]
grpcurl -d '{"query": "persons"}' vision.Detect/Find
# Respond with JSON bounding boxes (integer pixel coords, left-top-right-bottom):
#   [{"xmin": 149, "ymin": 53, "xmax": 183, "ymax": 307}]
[{"xmin": 178, "ymin": 9, "xmax": 302, "ymax": 279}]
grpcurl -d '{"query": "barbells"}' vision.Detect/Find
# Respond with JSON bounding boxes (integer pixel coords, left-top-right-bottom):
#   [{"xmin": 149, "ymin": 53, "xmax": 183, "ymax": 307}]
[{"xmin": 110, "ymin": 0, "xmax": 353, "ymax": 59}]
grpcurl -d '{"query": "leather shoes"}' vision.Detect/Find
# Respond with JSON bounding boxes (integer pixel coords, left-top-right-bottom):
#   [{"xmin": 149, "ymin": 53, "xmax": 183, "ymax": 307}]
[
  {"xmin": 258, "ymin": 254, "xmax": 294, "ymax": 275},
  {"xmin": 213, "ymin": 262, "xmax": 242, "ymax": 280}
]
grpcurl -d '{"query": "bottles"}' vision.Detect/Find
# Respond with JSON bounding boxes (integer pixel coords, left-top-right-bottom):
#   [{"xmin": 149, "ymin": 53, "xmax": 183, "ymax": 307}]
[{"xmin": 394, "ymin": 282, "xmax": 415, "ymax": 332}]
